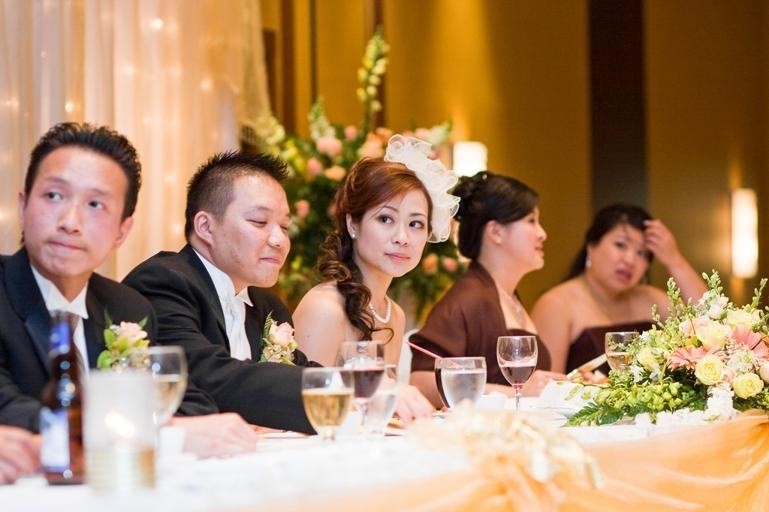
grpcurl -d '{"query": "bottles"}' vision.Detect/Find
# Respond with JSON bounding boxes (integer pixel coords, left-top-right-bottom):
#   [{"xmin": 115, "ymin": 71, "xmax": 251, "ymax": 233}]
[{"xmin": 35, "ymin": 308, "xmax": 88, "ymax": 482}]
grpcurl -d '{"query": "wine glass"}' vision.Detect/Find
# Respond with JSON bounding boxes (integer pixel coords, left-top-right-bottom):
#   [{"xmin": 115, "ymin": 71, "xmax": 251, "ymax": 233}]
[
  {"xmin": 496, "ymin": 335, "xmax": 539, "ymax": 407},
  {"xmin": 130, "ymin": 345, "xmax": 188, "ymax": 471}
]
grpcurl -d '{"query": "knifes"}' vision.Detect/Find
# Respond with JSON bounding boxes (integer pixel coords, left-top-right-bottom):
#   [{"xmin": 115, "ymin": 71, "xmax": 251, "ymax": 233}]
[{"xmin": 565, "ymin": 354, "xmax": 607, "ymax": 379}]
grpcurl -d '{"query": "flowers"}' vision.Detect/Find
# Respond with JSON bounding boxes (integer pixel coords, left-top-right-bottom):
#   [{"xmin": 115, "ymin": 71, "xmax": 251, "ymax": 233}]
[
  {"xmin": 228, "ymin": 24, "xmax": 495, "ymax": 310},
  {"xmin": 564, "ymin": 265, "xmax": 767, "ymax": 430},
  {"xmin": 258, "ymin": 318, "xmax": 299, "ymax": 366},
  {"xmin": 103, "ymin": 320, "xmax": 149, "ymax": 370}
]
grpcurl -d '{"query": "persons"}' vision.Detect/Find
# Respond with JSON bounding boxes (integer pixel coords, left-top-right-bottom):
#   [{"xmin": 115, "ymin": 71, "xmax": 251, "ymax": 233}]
[
  {"xmin": 122, "ymin": 148, "xmax": 433, "ymax": 436},
  {"xmin": 0, "ymin": 122, "xmax": 160, "ymax": 487},
  {"xmin": 407, "ymin": 169, "xmax": 608, "ymax": 407},
  {"xmin": 0, "ymin": 424, "xmax": 41, "ymax": 488},
  {"xmin": 530, "ymin": 200, "xmax": 710, "ymax": 375},
  {"xmin": 288, "ymin": 155, "xmax": 436, "ymax": 423}
]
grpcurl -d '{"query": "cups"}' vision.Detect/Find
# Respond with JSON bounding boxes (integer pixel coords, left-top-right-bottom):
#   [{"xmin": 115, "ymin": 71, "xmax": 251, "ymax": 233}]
[
  {"xmin": 302, "ymin": 366, "xmax": 355, "ymax": 441},
  {"xmin": 333, "ymin": 340, "xmax": 387, "ymax": 424},
  {"xmin": 435, "ymin": 357, "xmax": 477, "ymax": 408},
  {"xmin": 440, "ymin": 356, "xmax": 487, "ymax": 407},
  {"xmin": 354, "ymin": 363, "xmax": 400, "ymax": 437},
  {"xmin": 605, "ymin": 332, "xmax": 638, "ymax": 371},
  {"xmin": 79, "ymin": 369, "xmax": 155, "ymax": 492}
]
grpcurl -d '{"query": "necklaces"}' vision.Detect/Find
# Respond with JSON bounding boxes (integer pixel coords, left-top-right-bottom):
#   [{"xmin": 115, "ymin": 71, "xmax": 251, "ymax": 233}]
[
  {"xmin": 366, "ymin": 294, "xmax": 394, "ymax": 323},
  {"xmin": 498, "ymin": 285, "xmax": 522, "ymax": 316}
]
